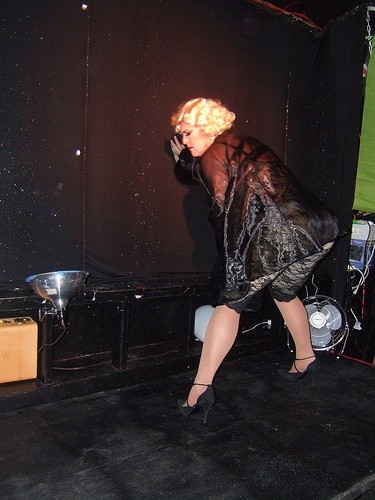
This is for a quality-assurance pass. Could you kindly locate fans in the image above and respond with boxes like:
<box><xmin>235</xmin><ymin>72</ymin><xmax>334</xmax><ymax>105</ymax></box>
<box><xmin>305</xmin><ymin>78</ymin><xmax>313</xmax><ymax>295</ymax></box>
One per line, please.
<box><xmin>286</xmin><ymin>294</ymin><xmax>351</xmax><ymax>359</ymax></box>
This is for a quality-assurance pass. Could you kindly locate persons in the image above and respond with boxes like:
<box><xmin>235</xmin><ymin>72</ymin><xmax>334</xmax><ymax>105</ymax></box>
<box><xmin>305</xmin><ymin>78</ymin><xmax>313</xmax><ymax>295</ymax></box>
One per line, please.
<box><xmin>168</xmin><ymin>96</ymin><xmax>354</xmax><ymax>425</ymax></box>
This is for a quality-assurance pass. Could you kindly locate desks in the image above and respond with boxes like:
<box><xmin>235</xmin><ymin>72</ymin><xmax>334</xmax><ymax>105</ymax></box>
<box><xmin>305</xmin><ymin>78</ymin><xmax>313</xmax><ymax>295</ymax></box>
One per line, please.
<box><xmin>0</xmin><ymin>273</ymin><xmax>281</xmax><ymax>387</ymax></box>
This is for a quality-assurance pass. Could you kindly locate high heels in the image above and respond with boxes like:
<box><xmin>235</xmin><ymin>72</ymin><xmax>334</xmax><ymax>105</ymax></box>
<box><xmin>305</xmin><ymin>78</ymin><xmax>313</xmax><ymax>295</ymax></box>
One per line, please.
<box><xmin>276</xmin><ymin>355</ymin><xmax>322</xmax><ymax>387</ymax></box>
<box><xmin>175</xmin><ymin>382</ymin><xmax>216</xmax><ymax>425</ymax></box>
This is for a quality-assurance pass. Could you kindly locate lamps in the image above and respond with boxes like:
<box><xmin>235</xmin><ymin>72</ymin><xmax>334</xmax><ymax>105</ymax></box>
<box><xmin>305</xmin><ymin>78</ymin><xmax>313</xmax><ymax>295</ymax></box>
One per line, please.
<box><xmin>25</xmin><ymin>270</ymin><xmax>90</xmax><ymax>355</ymax></box>
